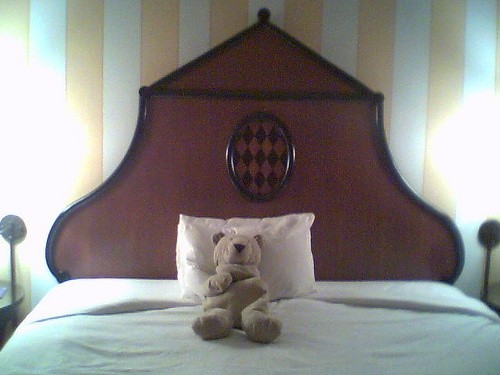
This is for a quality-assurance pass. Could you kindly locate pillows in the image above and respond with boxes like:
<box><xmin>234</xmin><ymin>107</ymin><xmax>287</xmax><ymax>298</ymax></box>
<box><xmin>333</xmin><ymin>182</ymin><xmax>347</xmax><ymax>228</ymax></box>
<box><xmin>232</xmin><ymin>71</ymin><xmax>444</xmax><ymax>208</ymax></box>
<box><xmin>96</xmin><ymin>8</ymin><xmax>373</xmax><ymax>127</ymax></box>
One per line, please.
<box><xmin>177</xmin><ymin>213</ymin><xmax>316</xmax><ymax>304</ymax></box>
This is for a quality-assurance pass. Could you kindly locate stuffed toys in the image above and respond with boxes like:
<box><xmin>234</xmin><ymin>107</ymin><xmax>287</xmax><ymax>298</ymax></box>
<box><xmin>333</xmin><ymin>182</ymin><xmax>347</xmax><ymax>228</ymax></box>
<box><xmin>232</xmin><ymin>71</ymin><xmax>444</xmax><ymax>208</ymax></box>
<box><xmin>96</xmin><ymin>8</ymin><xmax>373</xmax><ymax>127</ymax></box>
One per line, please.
<box><xmin>192</xmin><ymin>231</ymin><xmax>281</xmax><ymax>344</ymax></box>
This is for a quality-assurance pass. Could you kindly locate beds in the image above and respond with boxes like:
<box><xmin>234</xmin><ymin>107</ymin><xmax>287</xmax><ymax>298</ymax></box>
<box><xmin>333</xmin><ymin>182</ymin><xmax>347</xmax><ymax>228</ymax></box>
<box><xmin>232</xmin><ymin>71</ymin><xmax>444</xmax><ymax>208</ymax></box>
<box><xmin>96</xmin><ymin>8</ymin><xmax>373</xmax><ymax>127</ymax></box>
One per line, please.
<box><xmin>0</xmin><ymin>276</ymin><xmax>498</xmax><ymax>374</ymax></box>
